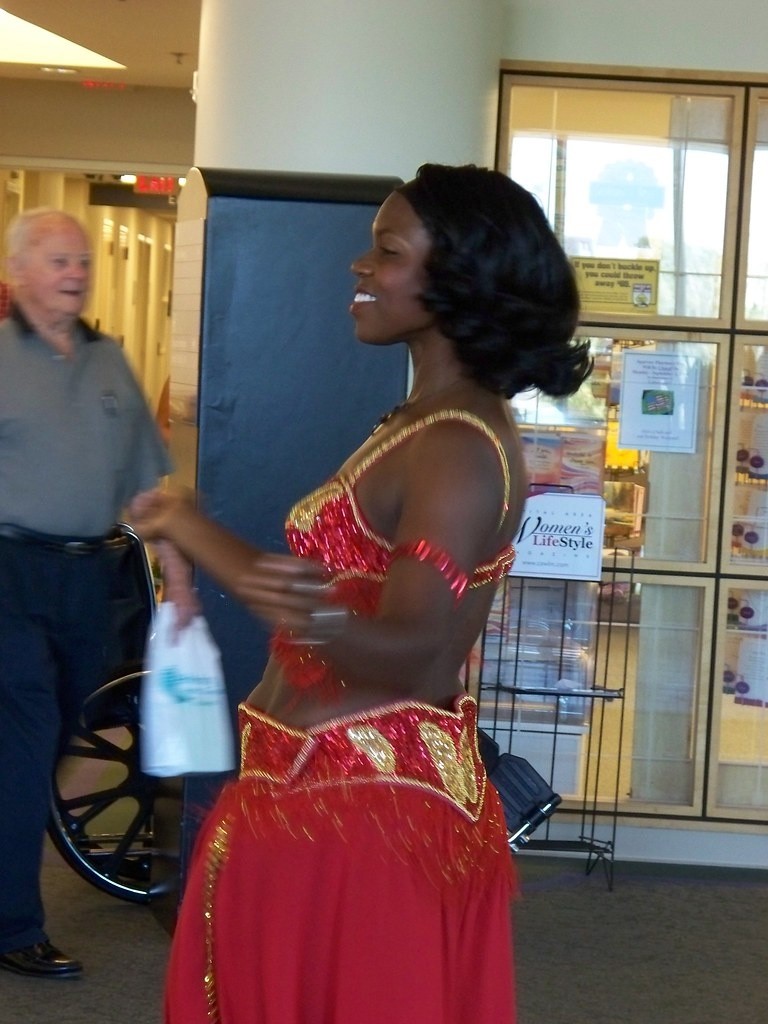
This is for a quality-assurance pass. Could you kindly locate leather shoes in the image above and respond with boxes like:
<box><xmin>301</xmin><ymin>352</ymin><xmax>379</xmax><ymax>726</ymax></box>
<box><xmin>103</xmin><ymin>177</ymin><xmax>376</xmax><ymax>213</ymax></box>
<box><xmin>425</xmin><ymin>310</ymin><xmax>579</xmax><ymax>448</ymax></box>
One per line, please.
<box><xmin>0</xmin><ymin>939</ymin><xmax>84</xmax><ymax>978</ymax></box>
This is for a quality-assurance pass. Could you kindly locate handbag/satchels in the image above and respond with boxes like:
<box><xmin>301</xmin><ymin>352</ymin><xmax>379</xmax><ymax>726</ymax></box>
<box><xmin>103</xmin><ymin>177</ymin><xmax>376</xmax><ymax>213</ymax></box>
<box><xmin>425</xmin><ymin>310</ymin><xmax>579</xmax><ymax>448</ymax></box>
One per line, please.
<box><xmin>139</xmin><ymin>602</ymin><xmax>235</xmax><ymax>777</ymax></box>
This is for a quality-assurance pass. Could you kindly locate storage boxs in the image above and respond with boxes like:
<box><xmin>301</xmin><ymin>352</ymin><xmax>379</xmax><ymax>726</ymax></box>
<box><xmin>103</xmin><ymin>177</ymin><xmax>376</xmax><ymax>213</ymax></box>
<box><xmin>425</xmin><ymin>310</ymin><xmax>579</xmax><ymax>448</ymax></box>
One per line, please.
<box><xmin>460</xmin><ymin>491</ymin><xmax>612</xmax><ymax>796</ymax></box>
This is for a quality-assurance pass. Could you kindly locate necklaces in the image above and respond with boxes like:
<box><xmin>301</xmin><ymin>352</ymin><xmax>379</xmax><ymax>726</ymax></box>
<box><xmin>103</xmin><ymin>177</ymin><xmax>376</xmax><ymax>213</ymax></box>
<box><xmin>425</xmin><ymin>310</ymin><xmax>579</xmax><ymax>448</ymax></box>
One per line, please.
<box><xmin>370</xmin><ymin>371</ymin><xmax>474</xmax><ymax>435</ymax></box>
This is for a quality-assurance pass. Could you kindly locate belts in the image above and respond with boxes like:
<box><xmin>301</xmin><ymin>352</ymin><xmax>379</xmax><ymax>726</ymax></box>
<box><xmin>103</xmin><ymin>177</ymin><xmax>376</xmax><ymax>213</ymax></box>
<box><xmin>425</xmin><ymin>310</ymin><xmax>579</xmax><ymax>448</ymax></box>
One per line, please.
<box><xmin>0</xmin><ymin>524</ymin><xmax>128</xmax><ymax>555</ymax></box>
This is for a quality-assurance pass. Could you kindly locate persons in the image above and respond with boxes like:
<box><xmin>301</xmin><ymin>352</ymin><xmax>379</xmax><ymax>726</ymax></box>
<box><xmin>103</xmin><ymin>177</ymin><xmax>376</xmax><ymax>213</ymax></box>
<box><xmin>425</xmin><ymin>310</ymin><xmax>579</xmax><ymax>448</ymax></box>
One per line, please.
<box><xmin>0</xmin><ymin>205</ymin><xmax>199</xmax><ymax>979</ymax></box>
<box><xmin>119</xmin><ymin>162</ymin><xmax>595</xmax><ymax>1024</ymax></box>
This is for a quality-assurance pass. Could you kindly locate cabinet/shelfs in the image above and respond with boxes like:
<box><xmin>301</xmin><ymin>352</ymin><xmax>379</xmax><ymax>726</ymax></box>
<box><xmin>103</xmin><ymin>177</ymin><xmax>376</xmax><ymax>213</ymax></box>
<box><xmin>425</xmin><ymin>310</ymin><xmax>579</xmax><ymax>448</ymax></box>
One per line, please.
<box><xmin>465</xmin><ymin>546</ymin><xmax>637</xmax><ymax>890</ymax></box>
<box><xmin>597</xmin><ymin>403</ymin><xmax>649</xmax><ymax>625</ymax></box>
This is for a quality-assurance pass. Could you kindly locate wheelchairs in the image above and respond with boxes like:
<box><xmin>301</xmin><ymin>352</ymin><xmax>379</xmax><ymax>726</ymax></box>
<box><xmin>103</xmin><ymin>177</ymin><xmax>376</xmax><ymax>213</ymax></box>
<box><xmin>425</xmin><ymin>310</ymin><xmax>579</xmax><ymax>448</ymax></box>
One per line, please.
<box><xmin>44</xmin><ymin>520</ymin><xmax>563</xmax><ymax>907</ymax></box>
